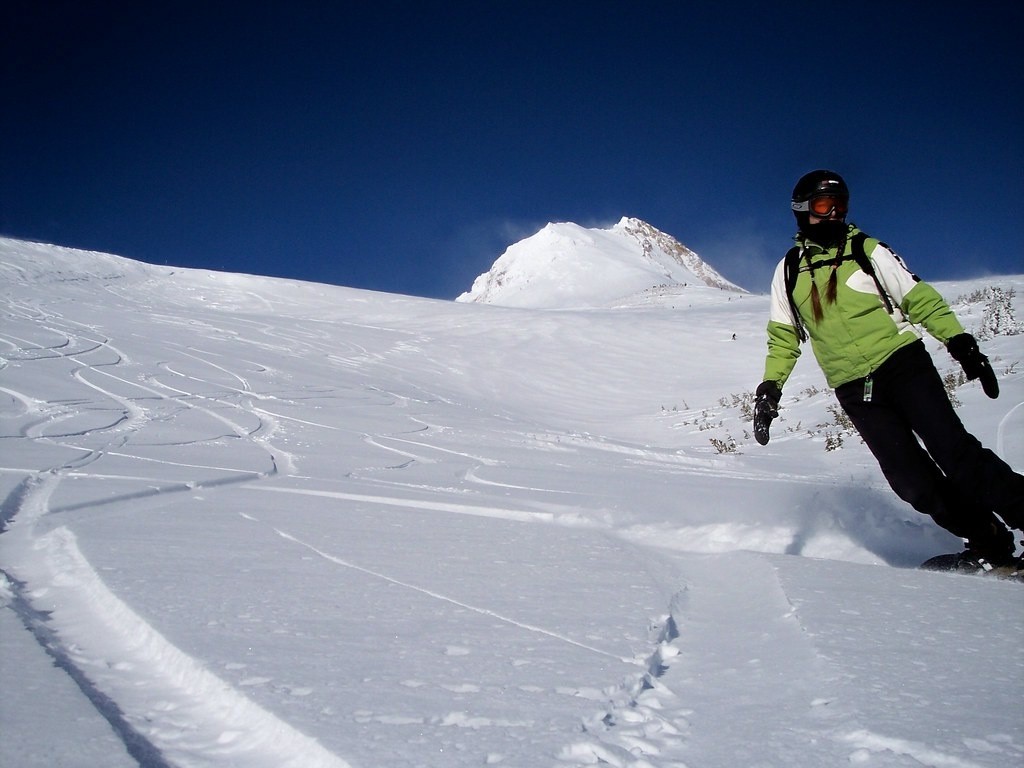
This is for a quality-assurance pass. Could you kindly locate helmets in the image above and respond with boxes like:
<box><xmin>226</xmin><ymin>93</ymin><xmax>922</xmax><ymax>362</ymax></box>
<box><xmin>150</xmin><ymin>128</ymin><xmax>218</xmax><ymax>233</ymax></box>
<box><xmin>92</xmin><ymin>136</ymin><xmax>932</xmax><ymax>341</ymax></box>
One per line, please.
<box><xmin>791</xmin><ymin>170</ymin><xmax>848</xmax><ymax>247</ymax></box>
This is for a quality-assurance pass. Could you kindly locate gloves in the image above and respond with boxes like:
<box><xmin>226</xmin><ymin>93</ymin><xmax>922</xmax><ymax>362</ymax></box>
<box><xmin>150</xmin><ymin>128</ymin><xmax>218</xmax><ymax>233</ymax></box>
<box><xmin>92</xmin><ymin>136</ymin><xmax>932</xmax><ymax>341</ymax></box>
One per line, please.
<box><xmin>753</xmin><ymin>380</ymin><xmax>782</xmax><ymax>445</ymax></box>
<box><xmin>947</xmin><ymin>333</ymin><xmax>999</xmax><ymax>399</ymax></box>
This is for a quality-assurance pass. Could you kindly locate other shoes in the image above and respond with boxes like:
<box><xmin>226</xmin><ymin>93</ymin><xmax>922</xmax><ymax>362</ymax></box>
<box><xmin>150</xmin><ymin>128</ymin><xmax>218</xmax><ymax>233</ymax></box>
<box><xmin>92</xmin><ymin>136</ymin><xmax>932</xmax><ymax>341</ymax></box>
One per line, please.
<box><xmin>952</xmin><ymin>549</ymin><xmax>1012</xmax><ymax>578</ymax></box>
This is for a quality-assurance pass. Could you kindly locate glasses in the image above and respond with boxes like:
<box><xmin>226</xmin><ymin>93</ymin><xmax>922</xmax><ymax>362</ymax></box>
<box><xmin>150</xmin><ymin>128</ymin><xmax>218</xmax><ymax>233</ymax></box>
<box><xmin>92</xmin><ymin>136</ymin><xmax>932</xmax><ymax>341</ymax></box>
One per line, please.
<box><xmin>791</xmin><ymin>193</ymin><xmax>849</xmax><ymax>218</ymax></box>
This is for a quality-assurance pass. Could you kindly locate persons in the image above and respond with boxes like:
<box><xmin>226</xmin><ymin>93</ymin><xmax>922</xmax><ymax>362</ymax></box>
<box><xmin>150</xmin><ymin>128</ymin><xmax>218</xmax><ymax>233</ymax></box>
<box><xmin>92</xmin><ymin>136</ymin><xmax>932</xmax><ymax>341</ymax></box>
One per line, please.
<box><xmin>754</xmin><ymin>169</ymin><xmax>1024</xmax><ymax>574</ymax></box>
